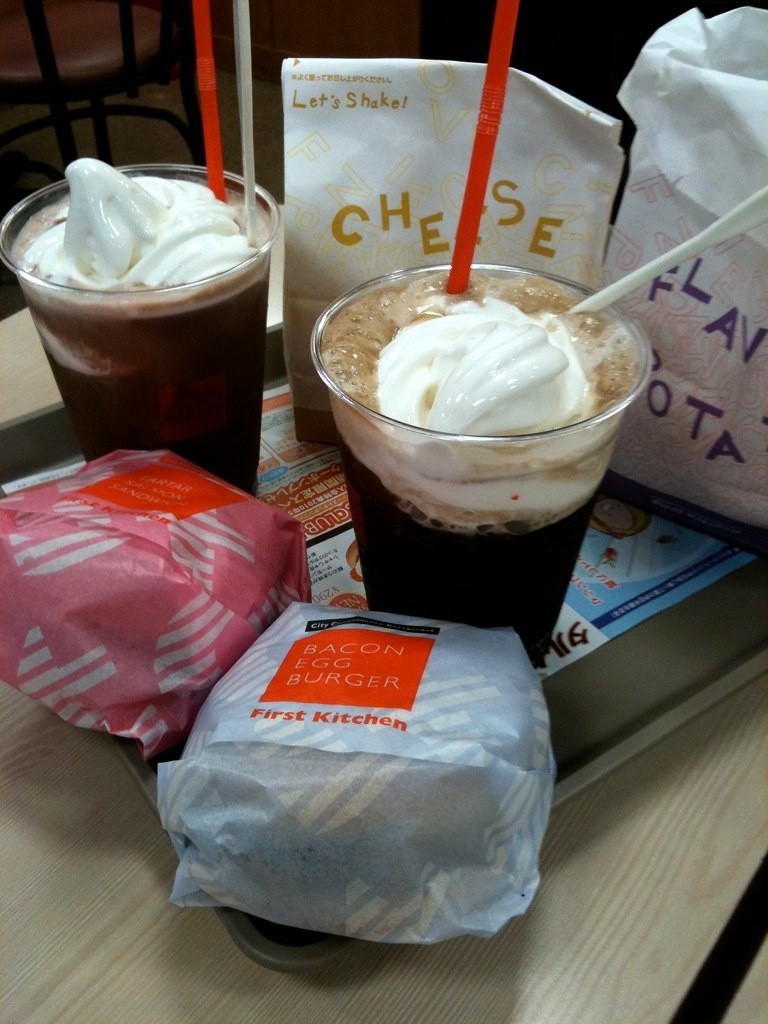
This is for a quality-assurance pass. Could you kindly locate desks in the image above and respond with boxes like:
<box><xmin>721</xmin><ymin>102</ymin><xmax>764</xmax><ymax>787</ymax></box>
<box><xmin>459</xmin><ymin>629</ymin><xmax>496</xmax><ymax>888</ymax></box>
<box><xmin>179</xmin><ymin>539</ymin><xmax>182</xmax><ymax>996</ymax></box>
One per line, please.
<box><xmin>0</xmin><ymin>202</ymin><xmax>768</xmax><ymax>1024</ymax></box>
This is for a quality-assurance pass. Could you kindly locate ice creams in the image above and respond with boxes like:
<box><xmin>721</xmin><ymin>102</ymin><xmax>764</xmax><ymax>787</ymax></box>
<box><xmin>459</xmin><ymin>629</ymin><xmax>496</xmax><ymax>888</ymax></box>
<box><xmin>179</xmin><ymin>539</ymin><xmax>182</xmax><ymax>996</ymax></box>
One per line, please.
<box><xmin>321</xmin><ymin>262</ymin><xmax>642</xmax><ymax>535</ymax></box>
<box><xmin>17</xmin><ymin>157</ymin><xmax>270</xmax><ymax>373</ymax></box>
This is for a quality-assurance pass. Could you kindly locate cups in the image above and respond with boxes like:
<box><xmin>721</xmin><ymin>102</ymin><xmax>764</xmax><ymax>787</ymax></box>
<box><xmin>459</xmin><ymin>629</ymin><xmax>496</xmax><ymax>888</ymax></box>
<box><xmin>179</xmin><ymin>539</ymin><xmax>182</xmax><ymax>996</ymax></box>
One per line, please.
<box><xmin>309</xmin><ymin>261</ymin><xmax>654</xmax><ymax>671</ymax></box>
<box><xmin>0</xmin><ymin>161</ymin><xmax>282</xmax><ymax>497</ymax></box>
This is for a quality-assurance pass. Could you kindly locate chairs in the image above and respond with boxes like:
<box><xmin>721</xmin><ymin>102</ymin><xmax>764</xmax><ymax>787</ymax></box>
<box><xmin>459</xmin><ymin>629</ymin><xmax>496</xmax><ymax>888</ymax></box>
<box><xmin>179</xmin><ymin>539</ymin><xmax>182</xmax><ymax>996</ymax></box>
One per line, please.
<box><xmin>0</xmin><ymin>0</ymin><xmax>207</xmax><ymax>188</ymax></box>
